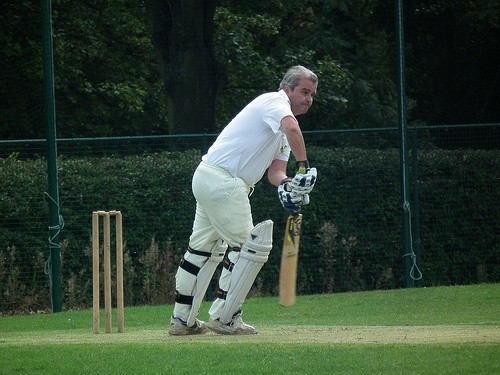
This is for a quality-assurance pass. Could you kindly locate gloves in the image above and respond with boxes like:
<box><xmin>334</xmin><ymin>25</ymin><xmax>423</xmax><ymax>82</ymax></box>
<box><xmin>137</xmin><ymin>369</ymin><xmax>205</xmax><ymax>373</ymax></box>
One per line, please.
<box><xmin>277</xmin><ymin>167</ymin><xmax>318</xmax><ymax>214</ymax></box>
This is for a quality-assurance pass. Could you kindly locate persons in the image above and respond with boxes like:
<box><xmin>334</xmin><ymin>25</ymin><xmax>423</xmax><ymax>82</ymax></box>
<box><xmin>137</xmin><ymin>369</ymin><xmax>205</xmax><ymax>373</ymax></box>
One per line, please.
<box><xmin>166</xmin><ymin>65</ymin><xmax>319</xmax><ymax>336</ymax></box>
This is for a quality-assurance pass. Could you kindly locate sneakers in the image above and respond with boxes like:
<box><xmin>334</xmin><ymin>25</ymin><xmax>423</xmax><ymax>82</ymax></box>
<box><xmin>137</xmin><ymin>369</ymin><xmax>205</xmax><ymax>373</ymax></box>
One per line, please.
<box><xmin>167</xmin><ymin>316</ymin><xmax>209</xmax><ymax>335</ymax></box>
<box><xmin>208</xmin><ymin>313</ymin><xmax>258</xmax><ymax>334</ymax></box>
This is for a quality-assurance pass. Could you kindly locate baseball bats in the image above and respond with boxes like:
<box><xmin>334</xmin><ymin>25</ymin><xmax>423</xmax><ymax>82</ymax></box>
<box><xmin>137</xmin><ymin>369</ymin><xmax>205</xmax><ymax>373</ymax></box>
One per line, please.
<box><xmin>279</xmin><ymin>166</ymin><xmax>306</xmax><ymax>308</ymax></box>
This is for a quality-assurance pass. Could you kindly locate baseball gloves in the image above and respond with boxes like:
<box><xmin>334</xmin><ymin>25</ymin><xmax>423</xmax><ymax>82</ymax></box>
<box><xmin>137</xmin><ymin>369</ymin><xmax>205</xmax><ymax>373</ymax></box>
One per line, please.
<box><xmin>278</xmin><ymin>160</ymin><xmax>317</xmax><ymax>213</ymax></box>
